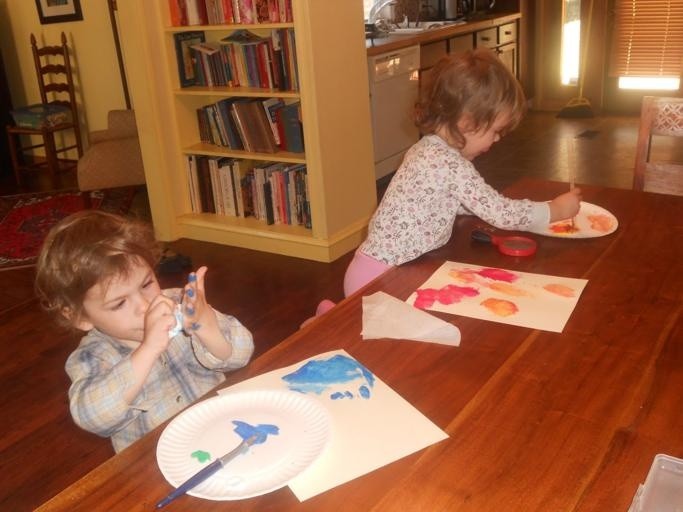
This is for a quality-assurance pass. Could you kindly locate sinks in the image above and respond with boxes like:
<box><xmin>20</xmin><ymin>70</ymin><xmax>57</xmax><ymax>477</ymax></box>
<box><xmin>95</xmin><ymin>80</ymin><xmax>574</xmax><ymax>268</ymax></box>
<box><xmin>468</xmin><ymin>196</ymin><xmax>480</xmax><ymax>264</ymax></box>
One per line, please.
<box><xmin>389</xmin><ymin>21</ymin><xmax>468</xmax><ymax>30</ymax></box>
<box><xmin>389</xmin><ymin>28</ymin><xmax>424</xmax><ymax>34</ymax></box>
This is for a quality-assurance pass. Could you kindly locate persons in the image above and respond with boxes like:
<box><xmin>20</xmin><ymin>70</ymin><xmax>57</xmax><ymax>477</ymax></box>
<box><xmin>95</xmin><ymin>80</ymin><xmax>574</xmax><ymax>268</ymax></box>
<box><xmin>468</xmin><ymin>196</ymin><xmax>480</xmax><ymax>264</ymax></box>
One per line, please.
<box><xmin>32</xmin><ymin>210</ymin><xmax>255</xmax><ymax>455</ymax></box>
<box><xmin>297</xmin><ymin>49</ymin><xmax>583</xmax><ymax>330</ymax></box>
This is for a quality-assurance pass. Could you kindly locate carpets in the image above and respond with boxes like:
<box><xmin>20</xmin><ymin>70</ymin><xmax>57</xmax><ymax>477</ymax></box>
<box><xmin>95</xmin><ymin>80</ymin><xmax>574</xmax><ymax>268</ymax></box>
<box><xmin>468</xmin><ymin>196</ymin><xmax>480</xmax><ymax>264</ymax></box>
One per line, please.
<box><xmin>0</xmin><ymin>183</ymin><xmax>136</xmax><ymax>271</ymax></box>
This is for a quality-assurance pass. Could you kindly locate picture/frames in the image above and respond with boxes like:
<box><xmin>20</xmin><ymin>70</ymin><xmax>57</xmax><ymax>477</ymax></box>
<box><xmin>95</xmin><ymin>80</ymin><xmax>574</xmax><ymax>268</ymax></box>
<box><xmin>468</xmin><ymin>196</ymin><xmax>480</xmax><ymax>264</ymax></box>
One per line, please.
<box><xmin>35</xmin><ymin>0</ymin><xmax>84</xmax><ymax>25</ymax></box>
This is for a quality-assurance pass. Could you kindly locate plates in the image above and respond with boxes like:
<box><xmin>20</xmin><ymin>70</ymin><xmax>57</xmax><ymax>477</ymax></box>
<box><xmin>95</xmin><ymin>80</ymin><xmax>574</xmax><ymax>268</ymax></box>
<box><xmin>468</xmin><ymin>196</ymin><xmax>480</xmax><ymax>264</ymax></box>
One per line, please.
<box><xmin>155</xmin><ymin>388</ymin><xmax>333</xmax><ymax>501</ymax></box>
<box><xmin>525</xmin><ymin>199</ymin><xmax>618</xmax><ymax>238</ymax></box>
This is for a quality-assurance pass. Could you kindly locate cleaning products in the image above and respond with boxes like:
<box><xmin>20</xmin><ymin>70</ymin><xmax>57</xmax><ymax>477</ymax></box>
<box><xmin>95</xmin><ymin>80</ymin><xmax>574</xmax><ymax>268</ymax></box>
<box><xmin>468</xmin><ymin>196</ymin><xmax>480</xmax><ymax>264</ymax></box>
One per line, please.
<box><xmin>444</xmin><ymin>0</ymin><xmax>457</xmax><ymax>19</ymax></box>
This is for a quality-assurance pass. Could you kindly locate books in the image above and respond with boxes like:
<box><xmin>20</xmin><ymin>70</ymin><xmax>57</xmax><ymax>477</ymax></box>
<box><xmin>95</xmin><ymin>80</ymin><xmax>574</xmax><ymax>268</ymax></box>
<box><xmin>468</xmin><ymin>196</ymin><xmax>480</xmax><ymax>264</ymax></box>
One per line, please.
<box><xmin>169</xmin><ymin>2</ymin><xmax>313</xmax><ymax>230</ymax></box>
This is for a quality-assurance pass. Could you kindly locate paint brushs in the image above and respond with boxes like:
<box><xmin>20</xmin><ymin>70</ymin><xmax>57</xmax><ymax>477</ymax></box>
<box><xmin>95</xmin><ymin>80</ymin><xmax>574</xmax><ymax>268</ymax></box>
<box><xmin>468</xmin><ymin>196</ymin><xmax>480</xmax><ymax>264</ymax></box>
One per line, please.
<box><xmin>157</xmin><ymin>434</ymin><xmax>258</xmax><ymax>508</ymax></box>
<box><xmin>567</xmin><ymin>136</ymin><xmax>575</xmax><ymax>228</ymax></box>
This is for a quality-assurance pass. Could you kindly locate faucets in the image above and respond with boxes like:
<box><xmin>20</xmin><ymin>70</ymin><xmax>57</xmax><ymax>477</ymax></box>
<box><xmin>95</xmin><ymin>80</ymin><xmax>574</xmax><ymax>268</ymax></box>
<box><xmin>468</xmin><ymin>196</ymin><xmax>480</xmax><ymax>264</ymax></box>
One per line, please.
<box><xmin>369</xmin><ymin>0</ymin><xmax>398</xmax><ymax>23</ymax></box>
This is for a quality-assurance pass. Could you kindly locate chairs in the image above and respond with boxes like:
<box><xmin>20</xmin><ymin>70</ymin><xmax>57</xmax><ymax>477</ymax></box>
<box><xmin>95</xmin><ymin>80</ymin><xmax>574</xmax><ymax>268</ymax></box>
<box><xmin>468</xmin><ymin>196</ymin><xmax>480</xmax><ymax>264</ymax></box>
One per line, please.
<box><xmin>632</xmin><ymin>95</ymin><xmax>683</xmax><ymax>197</ymax></box>
<box><xmin>8</xmin><ymin>32</ymin><xmax>84</xmax><ymax>189</ymax></box>
<box><xmin>77</xmin><ymin>109</ymin><xmax>145</xmax><ymax>209</ymax></box>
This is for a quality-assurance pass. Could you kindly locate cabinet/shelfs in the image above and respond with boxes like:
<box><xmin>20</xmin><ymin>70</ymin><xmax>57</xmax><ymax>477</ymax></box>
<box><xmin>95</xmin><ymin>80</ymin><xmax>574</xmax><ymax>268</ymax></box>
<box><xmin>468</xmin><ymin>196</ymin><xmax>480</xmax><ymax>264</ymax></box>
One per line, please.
<box><xmin>420</xmin><ymin>22</ymin><xmax>517</xmax><ymax>105</ymax></box>
<box><xmin>114</xmin><ymin>0</ymin><xmax>376</xmax><ymax>262</ymax></box>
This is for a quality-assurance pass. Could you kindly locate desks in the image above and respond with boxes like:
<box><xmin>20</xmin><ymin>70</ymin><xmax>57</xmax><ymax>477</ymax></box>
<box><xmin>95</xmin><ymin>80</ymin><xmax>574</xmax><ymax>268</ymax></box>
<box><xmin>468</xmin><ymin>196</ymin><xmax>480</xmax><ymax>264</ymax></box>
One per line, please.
<box><xmin>30</xmin><ymin>175</ymin><xmax>683</xmax><ymax>512</ymax></box>
